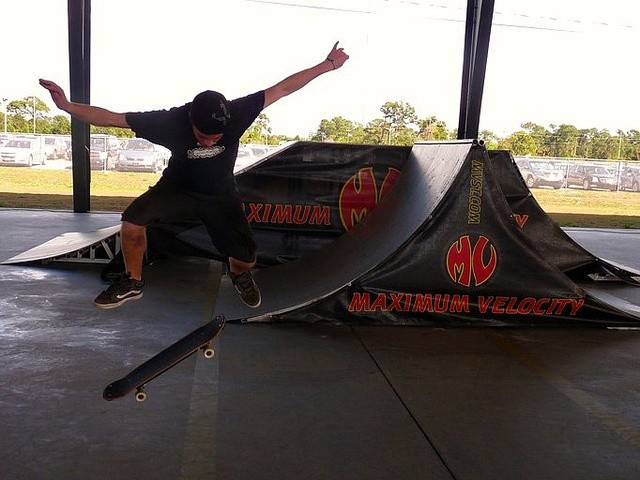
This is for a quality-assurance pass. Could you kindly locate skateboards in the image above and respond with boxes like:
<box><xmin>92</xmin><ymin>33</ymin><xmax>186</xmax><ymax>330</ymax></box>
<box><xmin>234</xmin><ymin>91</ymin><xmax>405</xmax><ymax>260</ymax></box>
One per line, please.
<box><xmin>103</xmin><ymin>314</ymin><xmax>226</xmax><ymax>402</ymax></box>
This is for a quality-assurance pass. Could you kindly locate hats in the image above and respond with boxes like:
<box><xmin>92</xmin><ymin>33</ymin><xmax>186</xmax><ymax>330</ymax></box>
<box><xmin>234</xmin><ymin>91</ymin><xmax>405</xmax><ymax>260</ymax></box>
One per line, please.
<box><xmin>191</xmin><ymin>90</ymin><xmax>232</xmax><ymax>136</ymax></box>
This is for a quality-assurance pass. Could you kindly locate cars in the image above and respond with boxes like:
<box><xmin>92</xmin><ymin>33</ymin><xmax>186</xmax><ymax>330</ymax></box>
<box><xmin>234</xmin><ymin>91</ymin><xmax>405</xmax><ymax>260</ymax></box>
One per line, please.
<box><xmin>235</xmin><ymin>147</ymin><xmax>266</xmax><ymax>166</ymax></box>
<box><xmin>619</xmin><ymin>165</ymin><xmax>640</xmax><ymax>192</ymax></box>
<box><xmin>566</xmin><ymin>164</ymin><xmax>618</xmax><ymax>191</ymax></box>
<box><xmin>554</xmin><ymin>162</ymin><xmax>567</xmax><ymax>178</ymax></box>
<box><xmin>114</xmin><ymin>138</ymin><xmax>163</xmax><ymax>173</ymax></box>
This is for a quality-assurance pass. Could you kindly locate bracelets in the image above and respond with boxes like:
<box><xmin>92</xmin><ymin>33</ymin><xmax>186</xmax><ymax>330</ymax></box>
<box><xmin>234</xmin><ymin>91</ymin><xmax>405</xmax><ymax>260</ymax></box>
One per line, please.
<box><xmin>326</xmin><ymin>57</ymin><xmax>335</xmax><ymax>70</ymax></box>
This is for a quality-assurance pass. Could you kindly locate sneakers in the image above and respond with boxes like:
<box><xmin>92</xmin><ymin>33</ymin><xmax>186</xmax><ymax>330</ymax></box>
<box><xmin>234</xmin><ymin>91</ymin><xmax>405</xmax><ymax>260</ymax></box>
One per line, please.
<box><xmin>93</xmin><ymin>271</ymin><xmax>145</xmax><ymax>310</ymax></box>
<box><xmin>226</xmin><ymin>265</ymin><xmax>261</xmax><ymax>308</ymax></box>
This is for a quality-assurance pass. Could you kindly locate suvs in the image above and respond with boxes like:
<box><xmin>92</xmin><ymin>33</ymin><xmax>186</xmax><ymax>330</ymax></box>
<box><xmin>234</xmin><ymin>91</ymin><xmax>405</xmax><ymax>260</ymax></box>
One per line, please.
<box><xmin>0</xmin><ymin>134</ymin><xmax>47</xmax><ymax>167</ymax></box>
<box><xmin>516</xmin><ymin>158</ymin><xmax>564</xmax><ymax>189</ymax></box>
<box><xmin>87</xmin><ymin>133</ymin><xmax>120</xmax><ymax>171</ymax></box>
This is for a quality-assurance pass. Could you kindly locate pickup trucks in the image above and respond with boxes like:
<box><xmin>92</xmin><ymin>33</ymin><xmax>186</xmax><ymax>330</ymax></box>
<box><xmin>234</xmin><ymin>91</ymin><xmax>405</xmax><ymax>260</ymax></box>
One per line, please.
<box><xmin>44</xmin><ymin>136</ymin><xmax>68</xmax><ymax>160</ymax></box>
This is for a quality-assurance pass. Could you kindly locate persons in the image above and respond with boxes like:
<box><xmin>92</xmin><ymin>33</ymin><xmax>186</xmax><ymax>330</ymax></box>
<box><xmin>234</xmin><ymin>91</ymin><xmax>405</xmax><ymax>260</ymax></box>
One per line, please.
<box><xmin>37</xmin><ymin>39</ymin><xmax>350</xmax><ymax>309</ymax></box>
